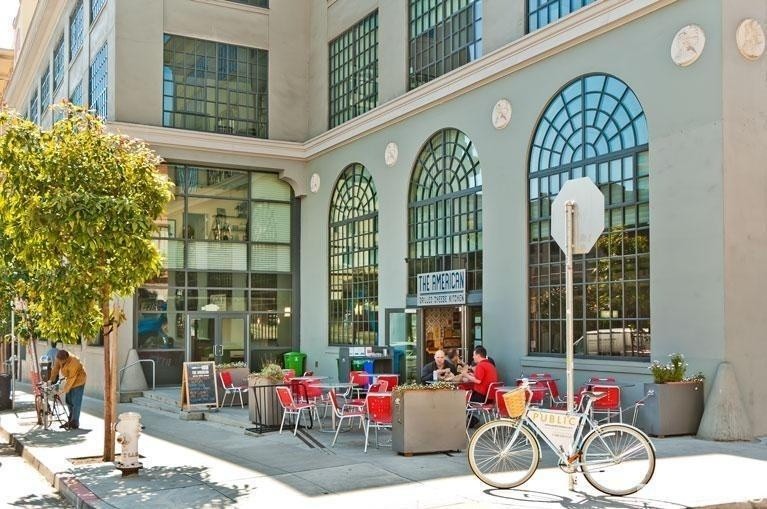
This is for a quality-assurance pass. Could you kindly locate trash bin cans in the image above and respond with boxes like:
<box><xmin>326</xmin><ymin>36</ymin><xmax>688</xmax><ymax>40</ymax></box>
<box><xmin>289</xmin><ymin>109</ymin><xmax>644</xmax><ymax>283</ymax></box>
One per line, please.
<box><xmin>282</xmin><ymin>352</ymin><xmax>307</xmax><ymax>376</ymax></box>
<box><xmin>0</xmin><ymin>372</ymin><xmax>12</xmax><ymax>409</ymax></box>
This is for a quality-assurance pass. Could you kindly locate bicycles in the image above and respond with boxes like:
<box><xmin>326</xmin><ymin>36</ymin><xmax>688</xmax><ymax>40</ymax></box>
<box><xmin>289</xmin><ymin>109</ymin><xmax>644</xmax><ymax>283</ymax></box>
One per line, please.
<box><xmin>464</xmin><ymin>377</ymin><xmax>656</xmax><ymax>498</ymax></box>
<box><xmin>34</xmin><ymin>375</ymin><xmax>71</xmax><ymax>432</ymax></box>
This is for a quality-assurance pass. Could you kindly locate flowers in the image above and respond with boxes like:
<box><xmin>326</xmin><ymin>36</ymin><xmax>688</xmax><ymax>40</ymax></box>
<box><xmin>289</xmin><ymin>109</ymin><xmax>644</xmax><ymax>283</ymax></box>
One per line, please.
<box><xmin>647</xmin><ymin>350</ymin><xmax>705</xmax><ymax>385</ymax></box>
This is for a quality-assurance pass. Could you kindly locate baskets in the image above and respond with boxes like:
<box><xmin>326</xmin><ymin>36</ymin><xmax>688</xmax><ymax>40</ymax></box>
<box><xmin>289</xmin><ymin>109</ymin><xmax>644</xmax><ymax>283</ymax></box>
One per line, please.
<box><xmin>501</xmin><ymin>386</ymin><xmax>527</xmax><ymax>418</ymax></box>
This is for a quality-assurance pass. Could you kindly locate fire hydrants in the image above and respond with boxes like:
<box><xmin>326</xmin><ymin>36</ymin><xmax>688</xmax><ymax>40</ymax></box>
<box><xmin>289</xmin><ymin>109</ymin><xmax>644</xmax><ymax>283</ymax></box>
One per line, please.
<box><xmin>110</xmin><ymin>411</ymin><xmax>145</xmax><ymax>478</ymax></box>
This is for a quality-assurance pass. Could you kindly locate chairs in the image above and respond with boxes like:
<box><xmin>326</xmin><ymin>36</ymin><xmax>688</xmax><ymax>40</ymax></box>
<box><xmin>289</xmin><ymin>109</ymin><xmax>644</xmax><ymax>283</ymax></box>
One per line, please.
<box><xmin>276</xmin><ymin>369</ymin><xmax>399</xmax><ymax>452</ymax></box>
<box><xmin>574</xmin><ymin>376</ymin><xmax>636</xmax><ymax>436</ymax></box>
<box><xmin>465</xmin><ymin>373</ymin><xmax>568</xmax><ymax>444</ymax></box>
<box><xmin>218</xmin><ymin>371</ymin><xmax>247</xmax><ymax>408</ymax></box>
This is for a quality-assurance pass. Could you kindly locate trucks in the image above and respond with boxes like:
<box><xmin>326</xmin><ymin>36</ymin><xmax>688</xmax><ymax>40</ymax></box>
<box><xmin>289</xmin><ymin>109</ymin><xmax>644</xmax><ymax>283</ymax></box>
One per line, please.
<box><xmin>573</xmin><ymin>328</ymin><xmax>632</xmax><ymax>356</ymax></box>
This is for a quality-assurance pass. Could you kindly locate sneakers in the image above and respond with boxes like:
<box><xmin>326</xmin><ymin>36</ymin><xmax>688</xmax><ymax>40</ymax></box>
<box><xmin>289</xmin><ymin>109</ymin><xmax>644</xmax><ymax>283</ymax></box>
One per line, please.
<box><xmin>59</xmin><ymin>420</ymin><xmax>80</xmax><ymax>432</ymax></box>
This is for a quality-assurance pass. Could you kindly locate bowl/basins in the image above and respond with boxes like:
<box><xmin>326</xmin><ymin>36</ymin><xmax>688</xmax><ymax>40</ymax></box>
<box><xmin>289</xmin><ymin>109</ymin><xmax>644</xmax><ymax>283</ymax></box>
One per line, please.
<box><xmin>463</xmin><ymin>378</ymin><xmax>470</xmax><ymax>382</ymax></box>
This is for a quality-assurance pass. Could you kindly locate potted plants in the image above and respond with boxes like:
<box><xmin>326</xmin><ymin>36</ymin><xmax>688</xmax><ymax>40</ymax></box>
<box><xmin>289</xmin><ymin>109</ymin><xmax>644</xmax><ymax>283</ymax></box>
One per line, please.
<box><xmin>215</xmin><ymin>360</ymin><xmax>249</xmax><ymax>405</ymax></box>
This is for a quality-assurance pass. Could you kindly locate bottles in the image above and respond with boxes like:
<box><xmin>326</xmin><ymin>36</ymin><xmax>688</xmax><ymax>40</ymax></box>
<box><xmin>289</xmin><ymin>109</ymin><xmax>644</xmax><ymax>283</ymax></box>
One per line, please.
<box><xmin>520</xmin><ymin>370</ymin><xmax>524</xmax><ymax>378</ymax></box>
<box><xmin>371</xmin><ymin>349</ymin><xmax>387</xmax><ymax>359</ymax></box>
<box><xmin>433</xmin><ymin>371</ymin><xmax>438</xmax><ymax>381</ymax></box>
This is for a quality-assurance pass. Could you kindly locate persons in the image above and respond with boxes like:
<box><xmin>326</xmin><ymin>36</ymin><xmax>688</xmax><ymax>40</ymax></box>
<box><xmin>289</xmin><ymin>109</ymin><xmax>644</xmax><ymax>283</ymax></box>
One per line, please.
<box><xmin>461</xmin><ymin>348</ymin><xmax>499</xmax><ymax>427</ymax></box>
<box><xmin>446</xmin><ymin>348</ymin><xmax>465</xmax><ymax>375</ymax></box>
<box><xmin>43</xmin><ymin>341</ymin><xmax>60</xmax><ymax>384</ymax></box>
<box><xmin>47</xmin><ymin>350</ymin><xmax>87</xmax><ymax>430</ymax></box>
<box><xmin>468</xmin><ymin>345</ymin><xmax>496</xmax><ymax>373</ymax></box>
<box><xmin>421</xmin><ymin>350</ymin><xmax>458</xmax><ymax>385</ymax></box>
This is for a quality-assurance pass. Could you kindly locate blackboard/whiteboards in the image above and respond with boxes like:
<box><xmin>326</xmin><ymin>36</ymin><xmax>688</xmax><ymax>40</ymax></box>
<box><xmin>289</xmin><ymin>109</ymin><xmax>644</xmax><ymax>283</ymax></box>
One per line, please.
<box><xmin>184</xmin><ymin>361</ymin><xmax>217</xmax><ymax>406</ymax></box>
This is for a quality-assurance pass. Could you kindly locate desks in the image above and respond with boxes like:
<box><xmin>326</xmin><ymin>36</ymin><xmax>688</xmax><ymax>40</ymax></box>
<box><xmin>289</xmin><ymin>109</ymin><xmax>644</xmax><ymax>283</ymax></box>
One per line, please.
<box><xmin>427</xmin><ymin>380</ymin><xmax>464</xmax><ymax>384</ymax></box>
